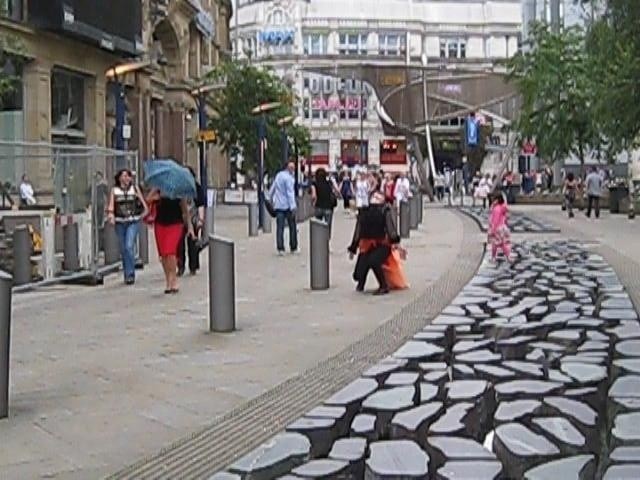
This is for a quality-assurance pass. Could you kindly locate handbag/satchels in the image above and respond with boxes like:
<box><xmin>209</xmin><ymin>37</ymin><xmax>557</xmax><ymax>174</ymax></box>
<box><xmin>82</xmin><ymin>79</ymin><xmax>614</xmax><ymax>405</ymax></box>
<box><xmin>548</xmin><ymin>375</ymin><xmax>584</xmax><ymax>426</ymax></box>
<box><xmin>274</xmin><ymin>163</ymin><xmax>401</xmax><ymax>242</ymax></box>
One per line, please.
<box><xmin>133</xmin><ymin>198</ymin><xmax>143</xmax><ymax>214</ymax></box>
<box><xmin>329</xmin><ymin>192</ymin><xmax>337</xmax><ymax>207</ymax></box>
<box><xmin>193</xmin><ymin>218</ymin><xmax>209</xmax><ymax>253</ymax></box>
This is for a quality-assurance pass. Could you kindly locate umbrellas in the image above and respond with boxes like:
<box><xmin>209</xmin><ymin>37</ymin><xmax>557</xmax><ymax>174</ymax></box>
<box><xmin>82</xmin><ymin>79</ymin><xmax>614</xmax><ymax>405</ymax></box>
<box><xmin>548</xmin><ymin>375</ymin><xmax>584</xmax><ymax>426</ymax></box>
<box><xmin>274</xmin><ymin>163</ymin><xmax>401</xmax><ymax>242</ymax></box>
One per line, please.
<box><xmin>143</xmin><ymin>158</ymin><xmax>197</xmax><ymax>199</ymax></box>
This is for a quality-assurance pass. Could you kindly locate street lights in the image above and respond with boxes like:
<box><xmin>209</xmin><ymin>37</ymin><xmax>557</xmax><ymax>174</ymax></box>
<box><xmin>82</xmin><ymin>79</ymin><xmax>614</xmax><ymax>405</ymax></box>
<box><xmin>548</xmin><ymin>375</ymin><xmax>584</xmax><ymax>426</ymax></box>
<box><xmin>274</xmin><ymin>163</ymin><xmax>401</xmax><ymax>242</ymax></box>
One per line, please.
<box><xmin>276</xmin><ymin>116</ymin><xmax>294</xmax><ymax>165</ymax></box>
<box><xmin>106</xmin><ymin>59</ymin><xmax>168</xmax><ymax>173</ymax></box>
<box><xmin>192</xmin><ymin>83</ymin><xmax>228</xmax><ymax>206</ymax></box>
<box><xmin>249</xmin><ymin>100</ymin><xmax>283</xmax><ymax>228</ymax></box>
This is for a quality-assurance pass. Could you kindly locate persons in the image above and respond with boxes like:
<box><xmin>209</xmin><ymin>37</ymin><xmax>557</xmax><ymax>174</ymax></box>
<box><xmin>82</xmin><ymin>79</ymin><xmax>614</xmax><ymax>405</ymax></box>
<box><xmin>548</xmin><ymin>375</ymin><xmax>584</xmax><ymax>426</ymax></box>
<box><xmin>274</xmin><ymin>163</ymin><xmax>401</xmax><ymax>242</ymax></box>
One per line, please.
<box><xmin>585</xmin><ymin>166</ymin><xmax>603</xmax><ymax>217</ymax></box>
<box><xmin>563</xmin><ymin>172</ymin><xmax>580</xmax><ymax>218</ymax></box>
<box><xmin>176</xmin><ymin>165</ymin><xmax>204</xmax><ymax>277</ymax></box>
<box><xmin>268</xmin><ymin>161</ymin><xmax>413</xmax><ymax>294</ymax></box>
<box><xmin>432</xmin><ymin>162</ymin><xmax>554</xmax><ymax>210</ymax></box>
<box><xmin>146</xmin><ymin>188</ymin><xmax>194</xmax><ymax>294</ymax></box>
<box><xmin>487</xmin><ymin>193</ymin><xmax>514</xmax><ymax>263</ymax></box>
<box><xmin>87</xmin><ymin>172</ymin><xmax>108</xmax><ymax>227</ymax></box>
<box><xmin>19</xmin><ymin>175</ymin><xmax>37</xmax><ymax>207</ymax></box>
<box><xmin>107</xmin><ymin>168</ymin><xmax>148</xmax><ymax>284</ymax></box>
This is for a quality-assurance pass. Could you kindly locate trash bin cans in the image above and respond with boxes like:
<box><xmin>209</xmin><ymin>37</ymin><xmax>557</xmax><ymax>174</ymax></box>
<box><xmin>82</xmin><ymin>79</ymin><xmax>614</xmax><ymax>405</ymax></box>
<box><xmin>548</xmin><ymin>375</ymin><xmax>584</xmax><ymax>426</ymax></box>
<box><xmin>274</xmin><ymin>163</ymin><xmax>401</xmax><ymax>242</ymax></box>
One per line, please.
<box><xmin>609</xmin><ymin>187</ymin><xmax>631</xmax><ymax>214</ymax></box>
